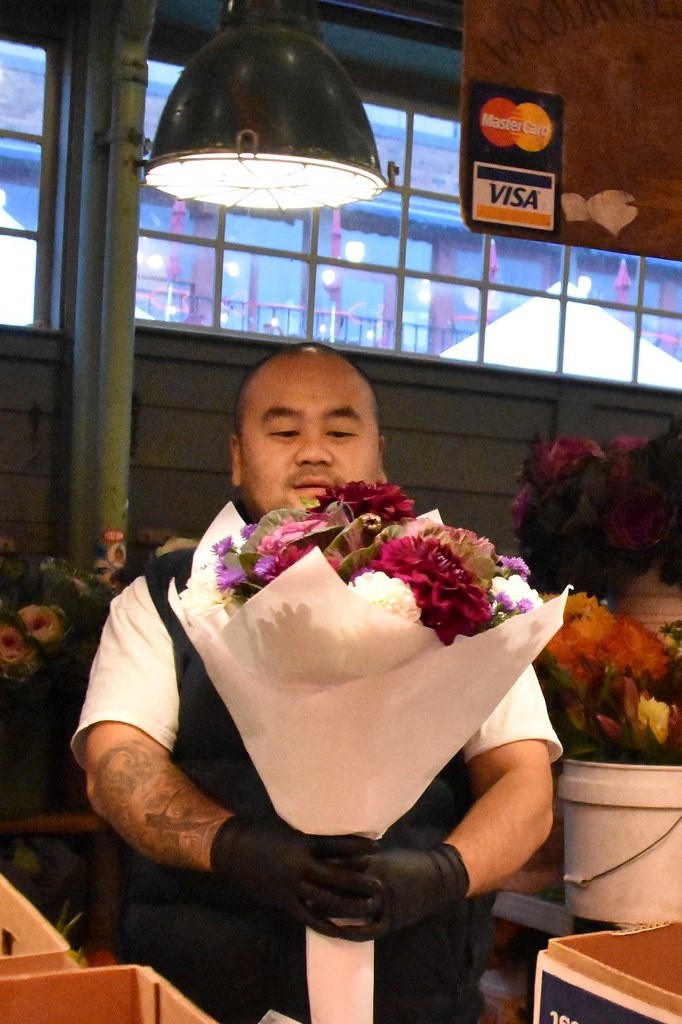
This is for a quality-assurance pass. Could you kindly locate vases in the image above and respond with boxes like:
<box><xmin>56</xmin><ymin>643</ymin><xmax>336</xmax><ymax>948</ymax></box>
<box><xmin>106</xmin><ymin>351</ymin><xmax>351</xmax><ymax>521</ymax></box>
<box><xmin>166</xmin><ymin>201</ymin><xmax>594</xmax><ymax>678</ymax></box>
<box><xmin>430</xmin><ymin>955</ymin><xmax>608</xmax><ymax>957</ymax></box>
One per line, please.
<box><xmin>558</xmin><ymin>757</ymin><xmax>681</xmax><ymax>922</ymax></box>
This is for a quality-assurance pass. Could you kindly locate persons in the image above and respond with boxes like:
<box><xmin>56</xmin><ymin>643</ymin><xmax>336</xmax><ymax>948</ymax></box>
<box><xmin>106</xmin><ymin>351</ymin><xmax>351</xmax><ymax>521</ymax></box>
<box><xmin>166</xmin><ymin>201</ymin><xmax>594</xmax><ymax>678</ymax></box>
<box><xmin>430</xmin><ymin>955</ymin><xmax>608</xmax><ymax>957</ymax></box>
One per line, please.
<box><xmin>69</xmin><ymin>340</ymin><xmax>566</xmax><ymax>1024</ymax></box>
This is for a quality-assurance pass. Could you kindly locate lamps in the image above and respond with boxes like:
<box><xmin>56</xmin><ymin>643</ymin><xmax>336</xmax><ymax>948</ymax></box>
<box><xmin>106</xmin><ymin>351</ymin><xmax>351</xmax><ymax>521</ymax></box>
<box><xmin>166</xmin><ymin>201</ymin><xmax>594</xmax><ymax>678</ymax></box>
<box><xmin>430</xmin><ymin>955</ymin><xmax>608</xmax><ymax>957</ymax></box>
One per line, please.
<box><xmin>147</xmin><ymin>1</ymin><xmax>385</xmax><ymax>207</ymax></box>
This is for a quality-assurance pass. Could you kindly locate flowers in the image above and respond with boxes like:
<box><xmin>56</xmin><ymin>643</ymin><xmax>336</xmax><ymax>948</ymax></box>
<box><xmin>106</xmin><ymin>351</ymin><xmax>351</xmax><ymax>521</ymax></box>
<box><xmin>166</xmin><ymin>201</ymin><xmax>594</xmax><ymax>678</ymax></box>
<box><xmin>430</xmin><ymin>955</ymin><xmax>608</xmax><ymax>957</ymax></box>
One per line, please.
<box><xmin>0</xmin><ymin>602</ymin><xmax>70</xmax><ymax>684</ymax></box>
<box><xmin>539</xmin><ymin>586</ymin><xmax>680</xmax><ymax>770</ymax></box>
<box><xmin>217</xmin><ymin>480</ymin><xmax>540</xmax><ymax>648</ymax></box>
<box><xmin>515</xmin><ymin>427</ymin><xmax>680</xmax><ymax>589</ymax></box>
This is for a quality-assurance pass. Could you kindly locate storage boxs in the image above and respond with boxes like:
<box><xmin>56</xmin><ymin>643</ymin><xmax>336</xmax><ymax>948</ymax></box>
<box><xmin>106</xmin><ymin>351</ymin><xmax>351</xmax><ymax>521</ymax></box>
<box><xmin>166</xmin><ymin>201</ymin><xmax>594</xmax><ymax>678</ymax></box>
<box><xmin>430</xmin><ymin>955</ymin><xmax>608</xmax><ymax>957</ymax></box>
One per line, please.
<box><xmin>1</xmin><ymin>965</ymin><xmax>220</xmax><ymax>1024</ymax></box>
<box><xmin>532</xmin><ymin>925</ymin><xmax>680</xmax><ymax>1024</ymax></box>
<box><xmin>1</xmin><ymin>874</ymin><xmax>68</xmax><ymax>974</ymax></box>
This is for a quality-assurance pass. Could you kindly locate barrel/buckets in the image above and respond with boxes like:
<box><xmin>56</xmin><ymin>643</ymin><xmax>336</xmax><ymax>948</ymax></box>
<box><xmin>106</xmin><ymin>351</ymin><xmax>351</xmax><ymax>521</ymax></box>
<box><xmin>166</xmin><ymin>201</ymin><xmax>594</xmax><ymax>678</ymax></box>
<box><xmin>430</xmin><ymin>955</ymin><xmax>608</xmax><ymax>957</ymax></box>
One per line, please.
<box><xmin>608</xmin><ymin>552</ymin><xmax>682</xmax><ymax>663</ymax></box>
<box><xmin>552</xmin><ymin>754</ymin><xmax>682</xmax><ymax>931</ymax></box>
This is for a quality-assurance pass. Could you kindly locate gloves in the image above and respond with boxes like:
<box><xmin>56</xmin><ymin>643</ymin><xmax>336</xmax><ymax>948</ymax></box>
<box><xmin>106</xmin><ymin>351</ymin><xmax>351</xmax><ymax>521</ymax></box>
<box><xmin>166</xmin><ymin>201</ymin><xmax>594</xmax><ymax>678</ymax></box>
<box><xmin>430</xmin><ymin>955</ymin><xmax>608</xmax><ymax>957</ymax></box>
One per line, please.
<box><xmin>311</xmin><ymin>842</ymin><xmax>470</xmax><ymax>941</ymax></box>
<box><xmin>211</xmin><ymin>814</ymin><xmax>385</xmax><ymax>938</ymax></box>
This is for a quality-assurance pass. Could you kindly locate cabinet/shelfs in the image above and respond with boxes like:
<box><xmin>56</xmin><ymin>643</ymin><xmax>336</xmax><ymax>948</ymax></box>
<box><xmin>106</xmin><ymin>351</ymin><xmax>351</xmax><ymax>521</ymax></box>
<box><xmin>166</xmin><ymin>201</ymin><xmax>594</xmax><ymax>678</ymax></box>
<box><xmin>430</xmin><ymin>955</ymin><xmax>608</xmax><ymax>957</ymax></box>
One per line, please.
<box><xmin>1</xmin><ymin>677</ymin><xmax>115</xmax><ymax>966</ymax></box>
